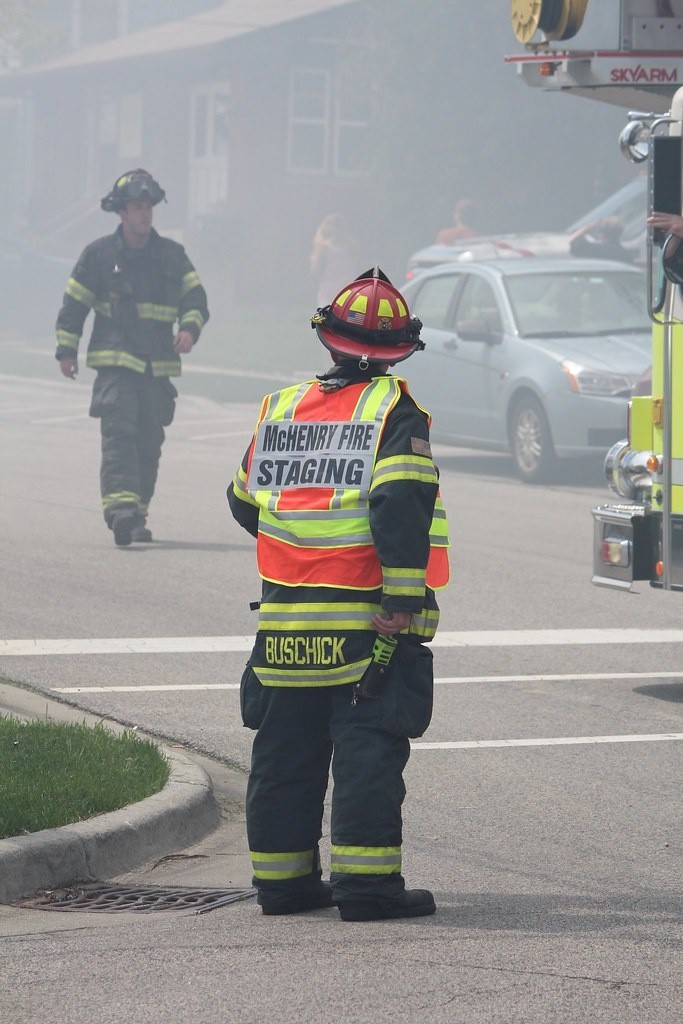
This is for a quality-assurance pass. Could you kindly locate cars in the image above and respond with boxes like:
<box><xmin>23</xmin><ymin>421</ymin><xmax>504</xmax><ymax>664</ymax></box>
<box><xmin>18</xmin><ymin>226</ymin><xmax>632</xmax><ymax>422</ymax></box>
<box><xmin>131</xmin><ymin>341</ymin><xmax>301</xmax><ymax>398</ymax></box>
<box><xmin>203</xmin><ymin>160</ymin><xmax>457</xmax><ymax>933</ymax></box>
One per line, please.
<box><xmin>385</xmin><ymin>258</ymin><xmax>652</xmax><ymax>484</ymax></box>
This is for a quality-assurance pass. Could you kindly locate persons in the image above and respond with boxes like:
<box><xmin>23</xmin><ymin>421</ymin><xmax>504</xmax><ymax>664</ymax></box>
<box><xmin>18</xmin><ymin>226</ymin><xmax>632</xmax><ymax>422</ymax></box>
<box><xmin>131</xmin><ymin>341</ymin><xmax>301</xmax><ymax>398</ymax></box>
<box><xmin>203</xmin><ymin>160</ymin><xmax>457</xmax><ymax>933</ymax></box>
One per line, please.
<box><xmin>54</xmin><ymin>169</ymin><xmax>210</xmax><ymax>547</ymax></box>
<box><xmin>435</xmin><ymin>200</ymin><xmax>485</xmax><ymax>246</ymax></box>
<box><xmin>312</xmin><ymin>213</ymin><xmax>355</xmax><ymax>308</ymax></box>
<box><xmin>226</xmin><ymin>265</ymin><xmax>451</xmax><ymax>921</ymax></box>
<box><xmin>645</xmin><ymin>211</ymin><xmax>683</xmax><ymax>237</ymax></box>
<box><xmin>568</xmin><ymin>215</ymin><xmax>639</xmax><ymax>263</ymax></box>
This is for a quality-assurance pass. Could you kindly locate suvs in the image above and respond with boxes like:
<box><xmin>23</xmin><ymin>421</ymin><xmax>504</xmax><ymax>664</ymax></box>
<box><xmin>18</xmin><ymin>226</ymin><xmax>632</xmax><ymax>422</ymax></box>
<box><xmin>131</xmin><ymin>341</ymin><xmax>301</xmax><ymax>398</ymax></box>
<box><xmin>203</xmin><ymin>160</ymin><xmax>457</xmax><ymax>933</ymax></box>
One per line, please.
<box><xmin>408</xmin><ymin>174</ymin><xmax>649</xmax><ymax>286</ymax></box>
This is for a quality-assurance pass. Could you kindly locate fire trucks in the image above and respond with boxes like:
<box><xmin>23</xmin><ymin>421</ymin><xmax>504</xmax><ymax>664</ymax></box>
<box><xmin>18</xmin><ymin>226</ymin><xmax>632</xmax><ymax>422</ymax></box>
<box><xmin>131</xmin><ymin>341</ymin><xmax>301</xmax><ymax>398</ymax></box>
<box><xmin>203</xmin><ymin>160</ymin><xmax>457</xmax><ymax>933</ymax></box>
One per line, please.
<box><xmin>503</xmin><ymin>1</ymin><xmax>683</xmax><ymax>595</ymax></box>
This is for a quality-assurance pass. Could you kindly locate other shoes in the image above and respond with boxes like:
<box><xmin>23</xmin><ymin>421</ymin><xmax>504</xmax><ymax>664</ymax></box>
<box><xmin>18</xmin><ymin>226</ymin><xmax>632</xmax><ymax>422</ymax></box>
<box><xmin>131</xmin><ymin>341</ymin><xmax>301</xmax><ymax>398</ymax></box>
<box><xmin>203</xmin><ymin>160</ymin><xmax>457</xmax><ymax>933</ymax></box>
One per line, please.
<box><xmin>261</xmin><ymin>879</ymin><xmax>337</xmax><ymax>914</ymax></box>
<box><xmin>337</xmin><ymin>889</ymin><xmax>436</xmax><ymax>921</ymax></box>
<box><xmin>112</xmin><ymin>512</ymin><xmax>132</xmax><ymax>546</ymax></box>
<box><xmin>127</xmin><ymin>517</ymin><xmax>152</xmax><ymax>542</ymax></box>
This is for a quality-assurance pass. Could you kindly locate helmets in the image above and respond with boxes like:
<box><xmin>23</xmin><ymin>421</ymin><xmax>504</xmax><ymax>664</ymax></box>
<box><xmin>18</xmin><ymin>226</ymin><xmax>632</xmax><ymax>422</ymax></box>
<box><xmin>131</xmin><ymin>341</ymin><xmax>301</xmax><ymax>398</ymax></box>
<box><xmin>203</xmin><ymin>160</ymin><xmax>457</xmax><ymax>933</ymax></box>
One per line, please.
<box><xmin>100</xmin><ymin>169</ymin><xmax>168</xmax><ymax>210</ymax></box>
<box><xmin>309</xmin><ymin>264</ymin><xmax>425</xmax><ymax>393</ymax></box>
